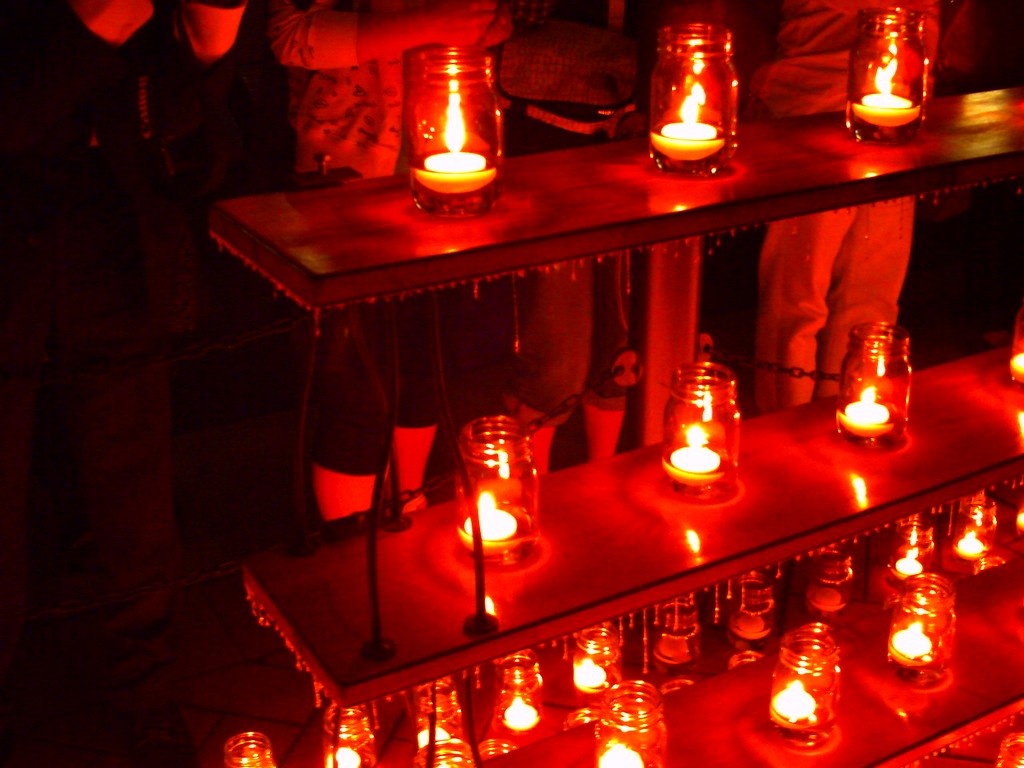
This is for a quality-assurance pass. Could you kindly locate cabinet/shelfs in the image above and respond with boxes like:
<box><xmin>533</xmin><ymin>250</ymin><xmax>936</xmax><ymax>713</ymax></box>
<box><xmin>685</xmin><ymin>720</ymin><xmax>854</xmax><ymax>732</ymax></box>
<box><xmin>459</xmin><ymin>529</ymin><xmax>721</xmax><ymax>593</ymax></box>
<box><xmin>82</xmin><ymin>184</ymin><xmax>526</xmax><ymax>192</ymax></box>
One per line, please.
<box><xmin>210</xmin><ymin>88</ymin><xmax>1024</xmax><ymax>768</ymax></box>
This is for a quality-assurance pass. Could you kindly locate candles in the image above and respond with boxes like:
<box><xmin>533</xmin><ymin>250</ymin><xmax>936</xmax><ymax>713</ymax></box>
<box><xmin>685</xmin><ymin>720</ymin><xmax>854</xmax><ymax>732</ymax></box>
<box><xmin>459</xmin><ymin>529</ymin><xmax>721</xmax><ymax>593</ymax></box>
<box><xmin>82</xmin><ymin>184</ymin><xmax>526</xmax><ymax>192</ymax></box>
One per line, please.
<box><xmin>324</xmin><ymin>60</ymin><xmax>996</xmax><ymax>768</ymax></box>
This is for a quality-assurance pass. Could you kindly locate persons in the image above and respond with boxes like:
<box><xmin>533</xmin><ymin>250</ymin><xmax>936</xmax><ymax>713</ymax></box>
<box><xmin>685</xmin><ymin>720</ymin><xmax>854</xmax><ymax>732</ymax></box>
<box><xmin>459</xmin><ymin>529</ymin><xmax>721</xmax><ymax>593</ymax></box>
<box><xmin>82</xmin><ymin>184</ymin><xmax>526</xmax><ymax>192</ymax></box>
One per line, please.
<box><xmin>265</xmin><ymin>0</ymin><xmax>1024</xmax><ymax>557</ymax></box>
<box><xmin>0</xmin><ymin>1</ymin><xmax>247</xmax><ymax>768</ymax></box>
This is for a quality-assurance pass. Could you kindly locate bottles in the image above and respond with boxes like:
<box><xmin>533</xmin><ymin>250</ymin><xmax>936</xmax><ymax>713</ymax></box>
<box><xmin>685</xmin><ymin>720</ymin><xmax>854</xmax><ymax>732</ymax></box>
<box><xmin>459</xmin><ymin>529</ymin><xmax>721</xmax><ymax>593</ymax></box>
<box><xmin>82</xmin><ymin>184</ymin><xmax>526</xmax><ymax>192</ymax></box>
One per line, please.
<box><xmin>454</xmin><ymin>415</ymin><xmax>538</xmax><ymax>561</ymax></box>
<box><xmin>407</xmin><ymin>47</ymin><xmax>504</xmax><ymax>218</ymax></box>
<box><xmin>650</xmin><ymin>24</ymin><xmax>739</xmax><ymax>176</ymax></box>
<box><xmin>663</xmin><ymin>363</ymin><xmax>743</xmax><ymax>486</ymax></box>
<box><xmin>726</xmin><ymin>548</ymin><xmax>856</xmax><ymax>746</ymax></box>
<box><xmin>847</xmin><ymin>9</ymin><xmax>930</xmax><ymax>144</ymax></box>
<box><xmin>886</xmin><ymin>496</ymin><xmax>998</xmax><ymax>670</ymax></box>
<box><xmin>837</xmin><ymin>321</ymin><xmax>911</xmax><ymax>447</ymax></box>
<box><xmin>572</xmin><ymin>599</ymin><xmax>702</xmax><ymax>768</ymax></box>
<box><xmin>223</xmin><ymin>732</ymin><xmax>278</xmax><ymax>768</ymax></box>
<box><xmin>415</xmin><ymin>655</ymin><xmax>542</xmax><ymax>768</ymax></box>
<box><xmin>321</xmin><ymin>708</ymin><xmax>379</xmax><ymax>768</ymax></box>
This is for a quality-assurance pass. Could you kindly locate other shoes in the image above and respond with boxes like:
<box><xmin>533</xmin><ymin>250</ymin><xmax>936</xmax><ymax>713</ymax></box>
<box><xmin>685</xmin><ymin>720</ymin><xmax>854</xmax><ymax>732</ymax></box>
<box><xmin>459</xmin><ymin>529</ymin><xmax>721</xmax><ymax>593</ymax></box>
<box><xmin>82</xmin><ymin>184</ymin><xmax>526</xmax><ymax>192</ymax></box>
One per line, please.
<box><xmin>125</xmin><ymin>705</ymin><xmax>196</xmax><ymax>768</ymax></box>
